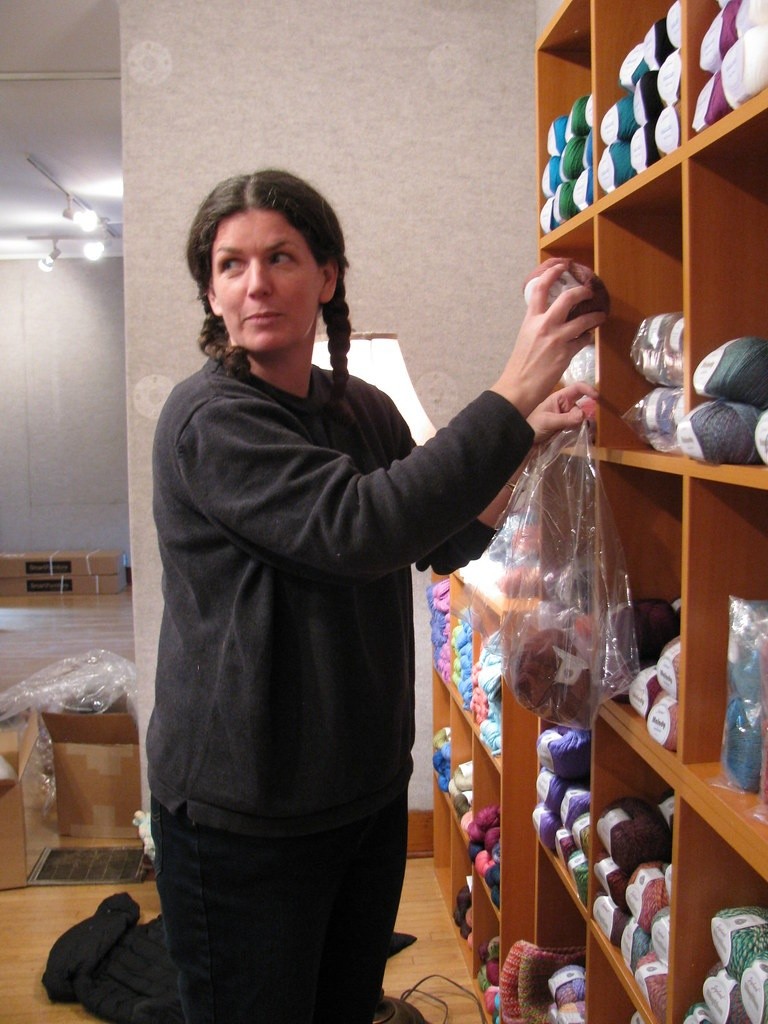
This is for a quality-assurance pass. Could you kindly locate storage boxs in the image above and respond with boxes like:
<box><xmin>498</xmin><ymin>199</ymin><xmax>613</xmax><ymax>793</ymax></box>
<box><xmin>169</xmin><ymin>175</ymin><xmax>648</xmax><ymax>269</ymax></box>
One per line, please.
<box><xmin>0</xmin><ymin>550</ymin><xmax>128</xmax><ymax>597</ymax></box>
<box><xmin>0</xmin><ymin>692</ymin><xmax>142</xmax><ymax>891</ymax></box>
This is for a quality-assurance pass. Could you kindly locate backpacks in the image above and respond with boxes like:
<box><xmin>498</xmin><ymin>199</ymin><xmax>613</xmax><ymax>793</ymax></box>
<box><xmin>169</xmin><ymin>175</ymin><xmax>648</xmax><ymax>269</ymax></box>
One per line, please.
<box><xmin>43</xmin><ymin>892</ymin><xmax>188</xmax><ymax>1024</ymax></box>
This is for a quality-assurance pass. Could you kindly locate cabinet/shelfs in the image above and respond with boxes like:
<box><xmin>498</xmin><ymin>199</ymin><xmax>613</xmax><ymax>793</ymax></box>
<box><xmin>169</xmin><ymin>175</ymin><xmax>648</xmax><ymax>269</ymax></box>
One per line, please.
<box><xmin>533</xmin><ymin>0</ymin><xmax>768</xmax><ymax>1024</ymax></box>
<box><xmin>430</xmin><ymin>557</ymin><xmax>538</xmax><ymax>1024</ymax></box>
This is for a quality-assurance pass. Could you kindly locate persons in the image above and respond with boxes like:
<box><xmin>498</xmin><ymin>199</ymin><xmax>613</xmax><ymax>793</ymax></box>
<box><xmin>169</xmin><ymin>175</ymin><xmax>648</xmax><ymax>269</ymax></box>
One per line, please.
<box><xmin>145</xmin><ymin>170</ymin><xmax>608</xmax><ymax>1024</ymax></box>
